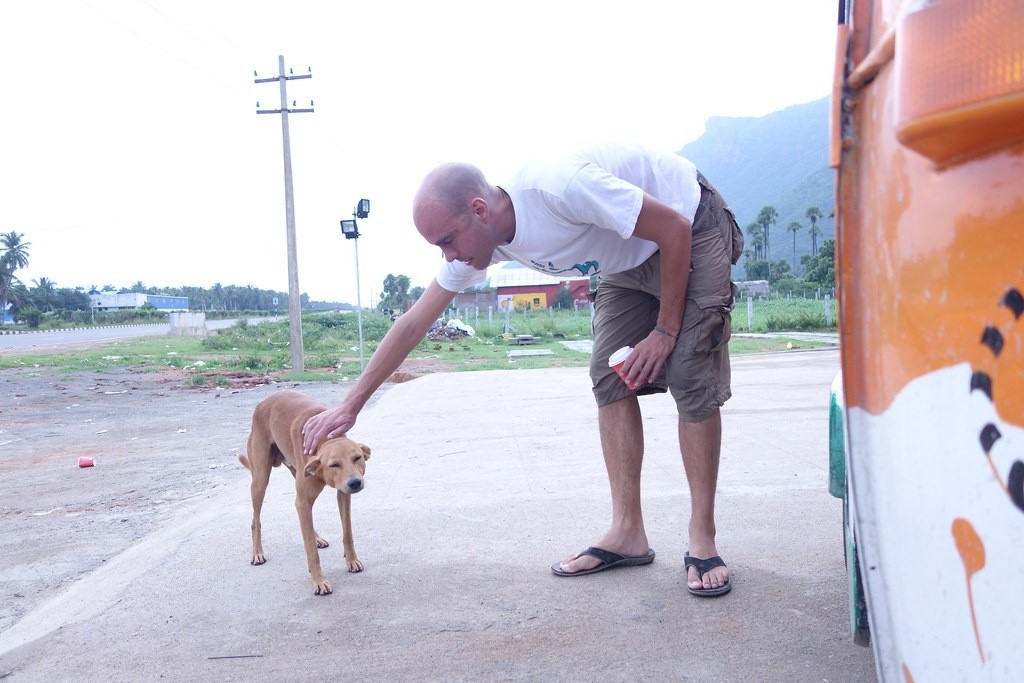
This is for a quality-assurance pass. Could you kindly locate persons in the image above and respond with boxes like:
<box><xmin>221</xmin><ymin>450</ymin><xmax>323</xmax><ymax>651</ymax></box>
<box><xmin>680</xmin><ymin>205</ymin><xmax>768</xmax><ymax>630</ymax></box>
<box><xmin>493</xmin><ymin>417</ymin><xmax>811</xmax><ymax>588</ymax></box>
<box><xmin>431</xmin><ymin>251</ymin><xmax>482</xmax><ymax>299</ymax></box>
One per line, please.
<box><xmin>301</xmin><ymin>147</ymin><xmax>745</xmax><ymax>599</ymax></box>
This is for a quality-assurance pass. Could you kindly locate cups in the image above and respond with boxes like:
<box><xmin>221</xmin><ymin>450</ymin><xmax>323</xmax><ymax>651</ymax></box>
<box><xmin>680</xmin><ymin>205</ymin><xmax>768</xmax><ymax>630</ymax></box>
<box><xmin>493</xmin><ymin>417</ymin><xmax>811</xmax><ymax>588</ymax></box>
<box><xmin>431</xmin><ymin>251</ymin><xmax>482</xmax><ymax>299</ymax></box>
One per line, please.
<box><xmin>609</xmin><ymin>345</ymin><xmax>646</xmax><ymax>390</ymax></box>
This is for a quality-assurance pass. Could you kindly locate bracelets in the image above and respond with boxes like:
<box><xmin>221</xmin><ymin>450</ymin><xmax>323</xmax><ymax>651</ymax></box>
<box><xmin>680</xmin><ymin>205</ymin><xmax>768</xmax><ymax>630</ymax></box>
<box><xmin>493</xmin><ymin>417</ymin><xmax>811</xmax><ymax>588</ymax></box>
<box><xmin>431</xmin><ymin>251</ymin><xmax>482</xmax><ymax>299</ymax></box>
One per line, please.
<box><xmin>653</xmin><ymin>324</ymin><xmax>678</xmax><ymax>341</ymax></box>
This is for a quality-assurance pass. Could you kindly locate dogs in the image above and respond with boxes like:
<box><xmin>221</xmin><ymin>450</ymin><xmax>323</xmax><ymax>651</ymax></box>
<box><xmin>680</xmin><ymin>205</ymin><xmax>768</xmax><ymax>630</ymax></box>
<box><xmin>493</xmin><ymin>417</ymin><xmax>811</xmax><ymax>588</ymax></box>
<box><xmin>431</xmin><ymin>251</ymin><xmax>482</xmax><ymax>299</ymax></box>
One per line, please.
<box><xmin>238</xmin><ymin>391</ymin><xmax>372</xmax><ymax>595</ymax></box>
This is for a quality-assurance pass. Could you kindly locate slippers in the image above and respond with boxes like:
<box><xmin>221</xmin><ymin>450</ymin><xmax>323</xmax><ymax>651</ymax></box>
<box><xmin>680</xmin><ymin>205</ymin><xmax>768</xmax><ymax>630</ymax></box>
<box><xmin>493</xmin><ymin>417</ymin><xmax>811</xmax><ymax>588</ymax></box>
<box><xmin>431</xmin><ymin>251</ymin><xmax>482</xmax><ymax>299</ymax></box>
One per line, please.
<box><xmin>684</xmin><ymin>550</ymin><xmax>734</xmax><ymax>598</ymax></box>
<box><xmin>551</xmin><ymin>544</ymin><xmax>656</xmax><ymax>576</ymax></box>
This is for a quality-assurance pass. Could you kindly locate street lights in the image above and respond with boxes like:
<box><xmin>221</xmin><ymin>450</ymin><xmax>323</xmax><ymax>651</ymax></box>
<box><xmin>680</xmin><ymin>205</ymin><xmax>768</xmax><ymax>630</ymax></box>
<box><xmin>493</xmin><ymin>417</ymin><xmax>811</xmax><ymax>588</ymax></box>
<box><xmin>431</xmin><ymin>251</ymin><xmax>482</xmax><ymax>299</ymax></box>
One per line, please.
<box><xmin>340</xmin><ymin>199</ymin><xmax>371</xmax><ymax>374</ymax></box>
